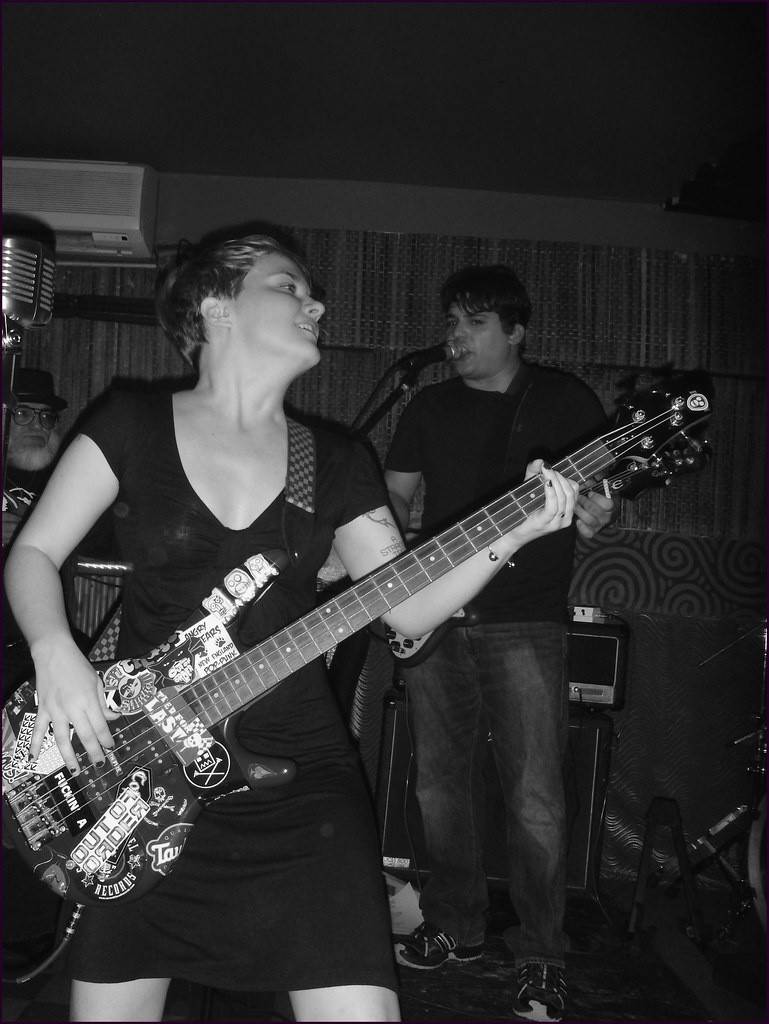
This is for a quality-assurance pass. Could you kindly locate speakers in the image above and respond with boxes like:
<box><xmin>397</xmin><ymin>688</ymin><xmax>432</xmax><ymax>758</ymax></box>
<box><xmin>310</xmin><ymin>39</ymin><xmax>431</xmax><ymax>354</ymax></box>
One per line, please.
<box><xmin>376</xmin><ymin>693</ymin><xmax>614</xmax><ymax>905</ymax></box>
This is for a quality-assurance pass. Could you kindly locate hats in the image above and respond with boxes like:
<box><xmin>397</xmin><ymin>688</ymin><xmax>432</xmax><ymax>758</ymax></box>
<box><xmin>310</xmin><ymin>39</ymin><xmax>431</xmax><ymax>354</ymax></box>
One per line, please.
<box><xmin>15</xmin><ymin>367</ymin><xmax>67</xmax><ymax>410</ymax></box>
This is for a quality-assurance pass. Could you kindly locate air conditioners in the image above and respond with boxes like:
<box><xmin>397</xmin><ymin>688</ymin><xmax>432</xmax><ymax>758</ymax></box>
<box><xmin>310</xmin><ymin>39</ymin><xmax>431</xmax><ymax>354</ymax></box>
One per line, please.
<box><xmin>2</xmin><ymin>157</ymin><xmax>160</xmax><ymax>260</ymax></box>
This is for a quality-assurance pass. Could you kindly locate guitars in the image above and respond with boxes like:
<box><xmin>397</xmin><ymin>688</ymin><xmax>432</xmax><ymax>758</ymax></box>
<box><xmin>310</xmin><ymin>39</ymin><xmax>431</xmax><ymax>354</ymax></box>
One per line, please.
<box><xmin>387</xmin><ymin>411</ymin><xmax>720</xmax><ymax>662</ymax></box>
<box><xmin>1</xmin><ymin>391</ymin><xmax>714</xmax><ymax>911</ymax></box>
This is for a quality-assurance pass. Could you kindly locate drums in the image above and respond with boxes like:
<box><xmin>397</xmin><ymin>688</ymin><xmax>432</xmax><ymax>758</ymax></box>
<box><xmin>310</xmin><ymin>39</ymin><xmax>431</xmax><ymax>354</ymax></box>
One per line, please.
<box><xmin>71</xmin><ymin>560</ymin><xmax>133</xmax><ymax>661</ymax></box>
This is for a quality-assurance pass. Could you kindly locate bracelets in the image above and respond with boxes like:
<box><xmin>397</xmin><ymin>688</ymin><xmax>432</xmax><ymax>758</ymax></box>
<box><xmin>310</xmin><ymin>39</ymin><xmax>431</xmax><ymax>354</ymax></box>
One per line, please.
<box><xmin>488</xmin><ymin>548</ymin><xmax>514</xmax><ymax>568</ymax></box>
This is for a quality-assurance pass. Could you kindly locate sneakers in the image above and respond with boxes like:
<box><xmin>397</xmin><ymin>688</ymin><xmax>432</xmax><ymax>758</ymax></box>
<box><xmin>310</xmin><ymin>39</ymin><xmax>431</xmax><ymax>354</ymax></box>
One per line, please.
<box><xmin>512</xmin><ymin>958</ymin><xmax>566</xmax><ymax>1023</ymax></box>
<box><xmin>392</xmin><ymin>921</ymin><xmax>486</xmax><ymax>970</ymax></box>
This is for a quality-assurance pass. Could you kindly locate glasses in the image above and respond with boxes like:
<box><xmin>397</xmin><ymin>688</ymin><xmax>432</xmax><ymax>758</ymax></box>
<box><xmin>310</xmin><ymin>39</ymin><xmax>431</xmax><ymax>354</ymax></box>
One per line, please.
<box><xmin>12</xmin><ymin>407</ymin><xmax>60</xmax><ymax>430</ymax></box>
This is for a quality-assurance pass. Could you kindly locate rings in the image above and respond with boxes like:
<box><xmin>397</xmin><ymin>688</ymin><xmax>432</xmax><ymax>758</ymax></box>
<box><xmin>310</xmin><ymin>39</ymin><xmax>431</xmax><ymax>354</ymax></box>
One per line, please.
<box><xmin>556</xmin><ymin>511</ymin><xmax>566</xmax><ymax>517</ymax></box>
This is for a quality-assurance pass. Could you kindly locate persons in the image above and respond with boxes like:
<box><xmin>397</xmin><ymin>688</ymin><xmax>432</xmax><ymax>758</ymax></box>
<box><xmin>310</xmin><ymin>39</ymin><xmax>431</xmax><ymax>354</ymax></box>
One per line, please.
<box><xmin>2</xmin><ymin>233</ymin><xmax>578</xmax><ymax>1022</ymax></box>
<box><xmin>383</xmin><ymin>268</ymin><xmax>614</xmax><ymax>1022</ymax></box>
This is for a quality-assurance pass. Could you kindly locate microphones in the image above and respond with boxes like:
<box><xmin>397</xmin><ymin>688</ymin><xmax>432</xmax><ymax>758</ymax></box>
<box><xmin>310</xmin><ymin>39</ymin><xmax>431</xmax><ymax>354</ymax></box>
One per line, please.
<box><xmin>1</xmin><ymin>236</ymin><xmax>56</xmax><ymax>352</ymax></box>
<box><xmin>397</xmin><ymin>340</ymin><xmax>461</xmax><ymax>370</ymax></box>
